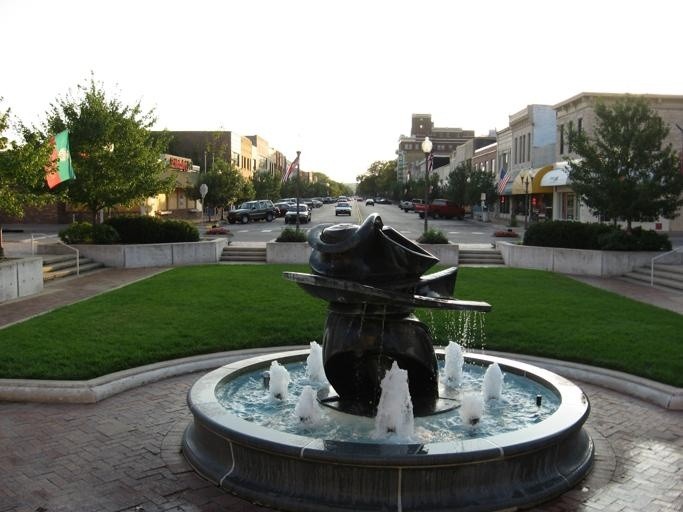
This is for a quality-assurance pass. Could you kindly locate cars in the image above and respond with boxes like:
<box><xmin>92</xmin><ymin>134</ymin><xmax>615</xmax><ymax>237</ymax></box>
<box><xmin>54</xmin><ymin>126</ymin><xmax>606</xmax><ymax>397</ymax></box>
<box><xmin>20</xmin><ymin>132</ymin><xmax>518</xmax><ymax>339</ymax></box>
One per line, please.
<box><xmin>380</xmin><ymin>199</ymin><xmax>388</xmax><ymax>202</ymax></box>
<box><xmin>275</xmin><ymin>195</ymin><xmax>374</xmax><ymax>224</ymax></box>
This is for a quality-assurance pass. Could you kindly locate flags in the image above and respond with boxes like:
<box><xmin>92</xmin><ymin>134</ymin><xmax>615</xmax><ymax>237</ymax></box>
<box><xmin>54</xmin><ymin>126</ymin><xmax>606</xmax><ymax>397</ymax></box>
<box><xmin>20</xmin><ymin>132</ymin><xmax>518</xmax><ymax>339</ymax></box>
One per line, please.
<box><xmin>280</xmin><ymin>155</ymin><xmax>299</xmax><ymax>183</ymax></box>
<box><xmin>495</xmin><ymin>167</ymin><xmax>510</xmax><ymax>196</ymax></box>
<box><xmin>39</xmin><ymin>128</ymin><xmax>77</xmax><ymax>191</ymax></box>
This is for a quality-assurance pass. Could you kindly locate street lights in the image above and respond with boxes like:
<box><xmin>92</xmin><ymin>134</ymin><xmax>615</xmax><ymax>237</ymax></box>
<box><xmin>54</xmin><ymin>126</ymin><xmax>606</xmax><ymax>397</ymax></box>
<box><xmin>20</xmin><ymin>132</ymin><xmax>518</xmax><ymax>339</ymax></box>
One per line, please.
<box><xmin>519</xmin><ymin>171</ymin><xmax>536</xmax><ymax>231</ymax></box>
<box><xmin>422</xmin><ymin>136</ymin><xmax>433</xmax><ymax>232</ymax></box>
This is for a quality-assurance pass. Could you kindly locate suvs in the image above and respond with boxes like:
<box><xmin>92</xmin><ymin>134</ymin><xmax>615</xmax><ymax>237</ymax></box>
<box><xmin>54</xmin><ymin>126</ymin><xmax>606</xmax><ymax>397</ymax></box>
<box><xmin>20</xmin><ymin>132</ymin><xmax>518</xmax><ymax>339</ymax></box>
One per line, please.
<box><xmin>226</xmin><ymin>199</ymin><xmax>275</xmax><ymax>224</ymax></box>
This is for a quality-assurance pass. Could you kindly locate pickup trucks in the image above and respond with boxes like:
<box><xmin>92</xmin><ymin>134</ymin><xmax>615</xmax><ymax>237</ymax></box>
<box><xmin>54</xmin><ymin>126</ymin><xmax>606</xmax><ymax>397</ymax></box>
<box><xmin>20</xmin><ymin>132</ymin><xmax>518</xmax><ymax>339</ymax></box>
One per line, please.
<box><xmin>401</xmin><ymin>199</ymin><xmax>425</xmax><ymax>213</ymax></box>
<box><xmin>415</xmin><ymin>199</ymin><xmax>466</xmax><ymax>220</ymax></box>
